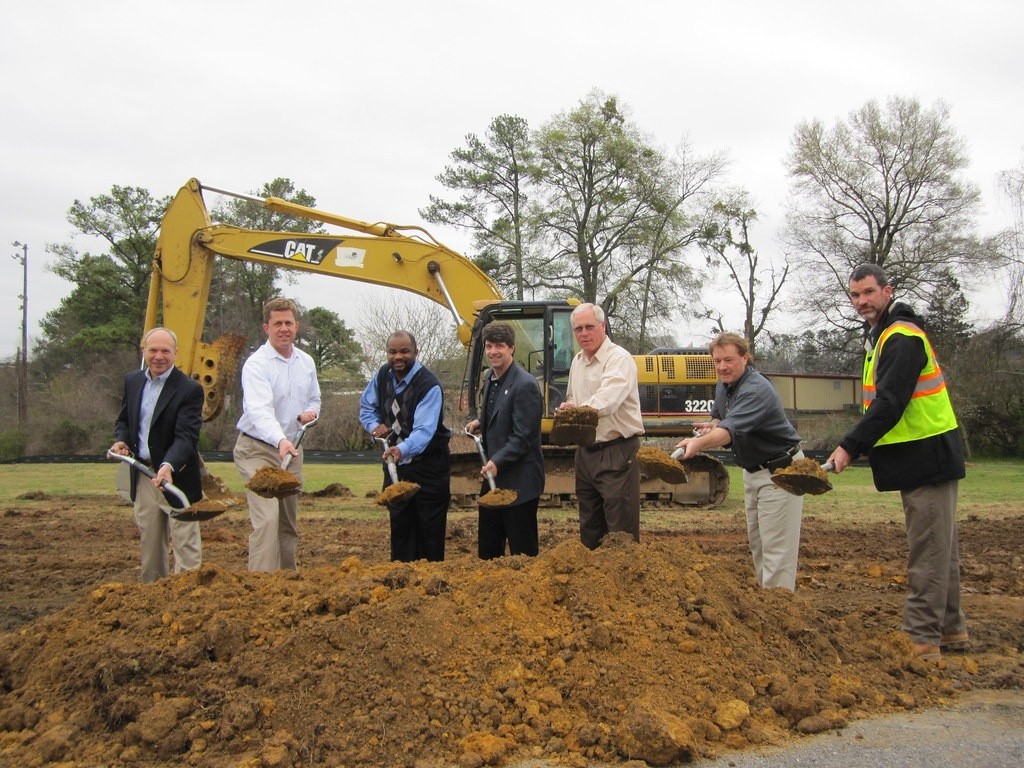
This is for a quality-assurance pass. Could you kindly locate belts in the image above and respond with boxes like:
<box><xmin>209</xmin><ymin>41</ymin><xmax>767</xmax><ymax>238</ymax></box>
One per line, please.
<box><xmin>587</xmin><ymin>435</ymin><xmax>625</xmax><ymax>453</ymax></box>
<box><xmin>136</xmin><ymin>459</ymin><xmax>154</xmax><ymax>467</ymax></box>
<box><xmin>243</xmin><ymin>432</ymin><xmax>273</xmax><ymax>447</ymax></box>
<box><xmin>745</xmin><ymin>443</ymin><xmax>803</xmax><ymax>473</ymax></box>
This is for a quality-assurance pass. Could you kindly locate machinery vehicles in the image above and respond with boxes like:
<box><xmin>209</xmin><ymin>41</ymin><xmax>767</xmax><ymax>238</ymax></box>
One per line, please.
<box><xmin>141</xmin><ymin>181</ymin><xmax>752</xmax><ymax>512</ymax></box>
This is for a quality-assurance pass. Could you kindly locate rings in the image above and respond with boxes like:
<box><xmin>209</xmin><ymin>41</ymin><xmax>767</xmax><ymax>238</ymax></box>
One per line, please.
<box><xmin>373</xmin><ymin>432</ymin><xmax>377</xmax><ymax>435</ymax></box>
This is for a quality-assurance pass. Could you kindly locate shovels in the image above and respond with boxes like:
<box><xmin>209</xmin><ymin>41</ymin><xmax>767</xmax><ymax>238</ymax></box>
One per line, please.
<box><xmin>638</xmin><ymin>426</ymin><xmax>711</xmax><ymax>484</ymax></box>
<box><xmin>464</xmin><ymin>426</ymin><xmax>519</xmax><ymax>508</ymax></box>
<box><xmin>771</xmin><ymin>461</ymin><xmax>834</xmax><ymax>497</ymax></box>
<box><xmin>550</xmin><ymin>409</ymin><xmax>600</xmax><ymax>448</ymax></box>
<box><xmin>372</xmin><ymin>426</ymin><xmax>420</xmax><ymax>505</ymax></box>
<box><xmin>251</xmin><ymin>413</ymin><xmax>320</xmax><ymax>498</ymax></box>
<box><xmin>106</xmin><ymin>448</ymin><xmax>226</xmax><ymax>521</ymax></box>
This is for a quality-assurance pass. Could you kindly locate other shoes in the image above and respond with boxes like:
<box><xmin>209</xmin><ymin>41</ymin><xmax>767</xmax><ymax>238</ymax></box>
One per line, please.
<box><xmin>940</xmin><ymin>631</ymin><xmax>971</xmax><ymax>650</ymax></box>
<box><xmin>908</xmin><ymin>638</ymin><xmax>942</xmax><ymax>659</ymax></box>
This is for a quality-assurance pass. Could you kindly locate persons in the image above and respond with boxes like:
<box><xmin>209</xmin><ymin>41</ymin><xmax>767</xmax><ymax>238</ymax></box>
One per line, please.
<box><xmin>359</xmin><ymin>329</ymin><xmax>454</xmax><ymax>564</ymax></box>
<box><xmin>233</xmin><ymin>297</ymin><xmax>322</xmax><ymax>573</ymax></box>
<box><xmin>107</xmin><ymin>327</ymin><xmax>205</xmax><ymax>586</ymax></box>
<box><xmin>826</xmin><ymin>264</ymin><xmax>966</xmax><ymax>659</ymax></box>
<box><xmin>464</xmin><ymin>321</ymin><xmax>545</xmax><ymax>560</ymax></box>
<box><xmin>560</xmin><ymin>302</ymin><xmax>645</xmax><ymax>551</ymax></box>
<box><xmin>676</xmin><ymin>332</ymin><xmax>805</xmax><ymax>592</ymax></box>
